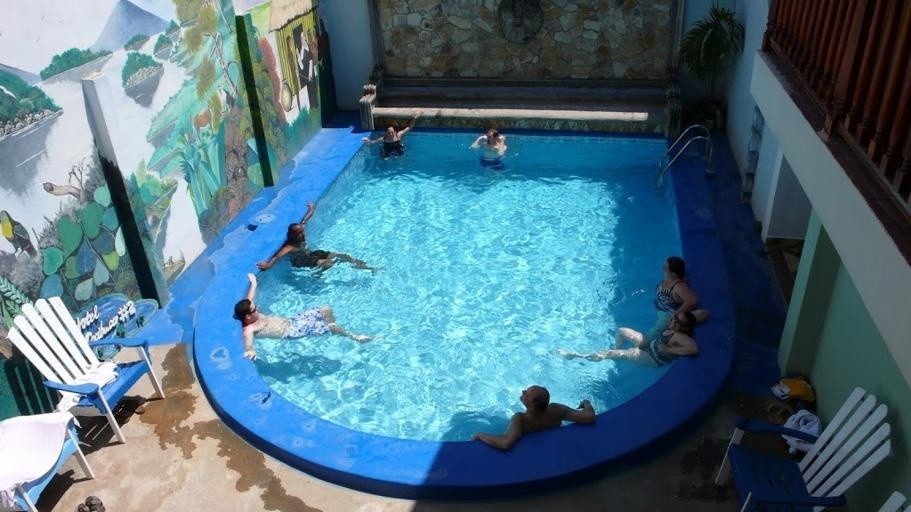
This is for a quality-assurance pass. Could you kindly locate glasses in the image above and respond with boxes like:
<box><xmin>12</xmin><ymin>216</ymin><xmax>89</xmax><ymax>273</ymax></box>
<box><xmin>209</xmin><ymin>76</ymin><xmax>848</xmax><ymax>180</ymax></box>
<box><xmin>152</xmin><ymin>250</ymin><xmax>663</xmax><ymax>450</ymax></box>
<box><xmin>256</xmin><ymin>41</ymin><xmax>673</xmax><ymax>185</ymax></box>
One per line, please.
<box><xmin>244</xmin><ymin>305</ymin><xmax>256</xmax><ymax>316</ymax></box>
<box><xmin>674</xmin><ymin>314</ymin><xmax>687</xmax><ymax>327</ymax></box>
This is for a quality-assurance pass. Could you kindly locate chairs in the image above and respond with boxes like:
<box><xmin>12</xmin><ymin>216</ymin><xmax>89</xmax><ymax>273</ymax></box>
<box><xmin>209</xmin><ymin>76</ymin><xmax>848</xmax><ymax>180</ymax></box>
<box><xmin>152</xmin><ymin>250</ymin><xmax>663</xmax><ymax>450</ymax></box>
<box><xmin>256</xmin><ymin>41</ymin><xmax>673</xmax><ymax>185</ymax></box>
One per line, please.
<box><xmin>713</xmin><ymin>387</ymin><xmax>895</xmax><ymax>510</ymax></box>
<box><xmin>0</xmin><ymin>297</ymin><xmax>168</xmax><ymax>512</ymax></box>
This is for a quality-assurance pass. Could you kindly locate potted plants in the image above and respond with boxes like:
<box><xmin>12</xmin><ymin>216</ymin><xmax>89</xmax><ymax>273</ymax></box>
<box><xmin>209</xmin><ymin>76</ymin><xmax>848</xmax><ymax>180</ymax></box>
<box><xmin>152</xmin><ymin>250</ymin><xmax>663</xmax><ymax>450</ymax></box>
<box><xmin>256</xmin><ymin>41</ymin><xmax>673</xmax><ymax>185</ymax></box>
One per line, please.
<box><xmin>677</xmin><ymin>3</ymin><xmax>746</xmax><ymax>134</ymax></box>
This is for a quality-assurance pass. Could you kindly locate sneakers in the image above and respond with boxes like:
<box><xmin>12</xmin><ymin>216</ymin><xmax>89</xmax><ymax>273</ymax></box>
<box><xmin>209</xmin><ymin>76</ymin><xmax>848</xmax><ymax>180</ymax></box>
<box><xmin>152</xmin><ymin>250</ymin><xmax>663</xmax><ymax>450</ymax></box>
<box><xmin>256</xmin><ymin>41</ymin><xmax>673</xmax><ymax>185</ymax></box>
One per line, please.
<box><xmin>125</xmin><ymin>401</ymin><xmax>145</xmax><ymax>414</ymax></box>
<box><xmin>115</xmin><ymin>414</ymin><xmax>130</xmax><ymax>424</ymax></box>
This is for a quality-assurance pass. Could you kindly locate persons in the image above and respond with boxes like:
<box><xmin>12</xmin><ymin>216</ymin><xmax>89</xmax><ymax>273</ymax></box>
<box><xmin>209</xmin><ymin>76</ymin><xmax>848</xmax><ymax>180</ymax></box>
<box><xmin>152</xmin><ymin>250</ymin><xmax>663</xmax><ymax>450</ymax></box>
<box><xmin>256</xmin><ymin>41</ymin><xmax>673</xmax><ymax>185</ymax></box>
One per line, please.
<box><xmin>362</xmin><ymin>113</ymin><xmax>421</xmax><ymax>159</ymax></box>
<box><xmin>255</xmin><ymin>203</ymin><xmax>376</xmax><ymax>277</ymax></box>
<box><xmin>1</xmin><ymin>108</ymin><xmax>57</xmax><ymax>139</ymax></box>
<box><xmin>557</xmin><ymin>309</ymin><xmax>709</xmax><ymax>368</ymax></box>
<box><xmin>234</xmin><ymin>271</ymin><xmax>374</xmax><ymax>362</ymax></box>
<box><xmin>286</xmin><ymin>19</ymin><xmax>336</xmax><ymax>117</ymax></box>
<box><xmin>471</xmin><ymin>129</ymin><xmax>508</xmax><ymax>172</ymax></box>
<box><xmin>472</xmin><ymin>385</ymin><xmax>596</xmax><ymax>450</ymax></box>
<box><xmin>653</xmin><ymin>256</ymin><xmax>700</xmax><ymax>333</ymax></box>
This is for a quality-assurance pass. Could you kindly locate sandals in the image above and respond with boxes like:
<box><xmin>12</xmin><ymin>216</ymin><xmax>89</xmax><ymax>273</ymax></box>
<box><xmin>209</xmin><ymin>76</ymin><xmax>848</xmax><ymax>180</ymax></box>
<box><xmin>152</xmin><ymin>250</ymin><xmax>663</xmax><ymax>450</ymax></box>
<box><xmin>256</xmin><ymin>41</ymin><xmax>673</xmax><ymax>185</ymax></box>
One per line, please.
<box><xmin>78</xmin><ymin>496</ymin><xmax>105</xmax><ymax>512</ymax></box>
